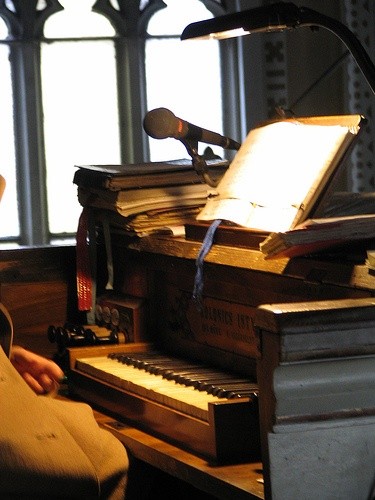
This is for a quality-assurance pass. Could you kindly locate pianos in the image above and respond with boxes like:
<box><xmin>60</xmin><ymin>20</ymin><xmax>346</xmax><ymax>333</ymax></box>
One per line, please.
<box><xmin>65</xmin><ymin>341</ymin><xmax>263</xmax><ymax>466</ymax></box>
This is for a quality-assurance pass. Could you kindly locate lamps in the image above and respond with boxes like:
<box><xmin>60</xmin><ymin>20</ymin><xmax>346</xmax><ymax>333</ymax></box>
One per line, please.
<box><xmin>181</xmin><ymin>2</ymin><xmax>375</xmax><ymax>97</ymax></box>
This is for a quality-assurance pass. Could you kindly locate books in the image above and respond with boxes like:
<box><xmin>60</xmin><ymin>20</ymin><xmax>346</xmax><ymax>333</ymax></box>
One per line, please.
<box><xmin>73</xmin><ymin>158</ymin><xmax>230</xmax><ymax>240</ymax></box>
<box><xmin>197</xmin><ymin>121</ymin><xmax>357</xmax><ymax>235</ymax></box>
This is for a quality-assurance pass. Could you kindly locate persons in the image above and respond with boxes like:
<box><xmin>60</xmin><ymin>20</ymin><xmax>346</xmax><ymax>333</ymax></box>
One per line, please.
<box><xmin>0</xmin><ymin>303</ymin><xmax>132</xmax><ymax>500</ymax></box>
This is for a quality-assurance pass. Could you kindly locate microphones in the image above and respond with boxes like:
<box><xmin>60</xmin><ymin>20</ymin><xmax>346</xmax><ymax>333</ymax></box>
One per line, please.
<box><xmin>143</xmin><ymin>107</ymin><xmax>241</xmax><ymax>151</ymax></box>
